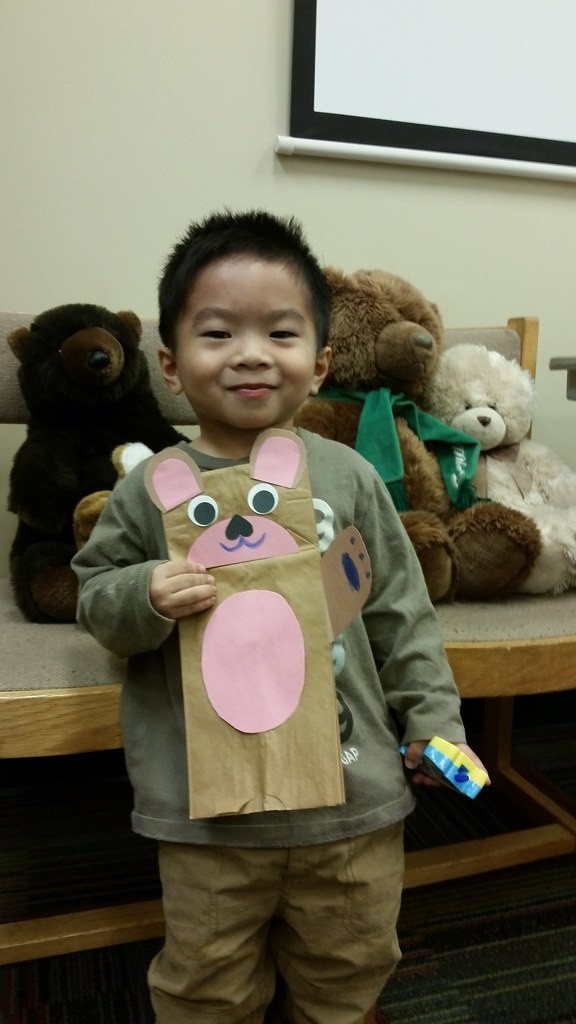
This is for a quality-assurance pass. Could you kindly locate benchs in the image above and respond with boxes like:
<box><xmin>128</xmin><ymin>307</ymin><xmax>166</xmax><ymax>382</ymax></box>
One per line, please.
<box><xmin>0</xmin><ymin>314</ymin><xmax>576</xmax><ymax>967</ymax></box>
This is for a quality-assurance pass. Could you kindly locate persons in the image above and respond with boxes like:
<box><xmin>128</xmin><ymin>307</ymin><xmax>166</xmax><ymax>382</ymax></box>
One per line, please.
<box><xmin>69</xmin><ymin>210</ymin><xmax>491</xmax><ymax>1024</ymax></box>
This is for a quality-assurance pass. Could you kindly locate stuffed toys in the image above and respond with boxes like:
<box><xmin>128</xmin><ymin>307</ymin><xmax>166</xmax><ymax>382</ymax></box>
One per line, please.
<box><xmin>6</xmin><ymin>303</ymin><xmax>195</xmax><ymax>626</ymax></box>
<box><xmin>422</xmin><ymin>344</ymin><xmax>576</xmax><ymax>596</ymax></box>
<box><xmin>292</xmin><ymin>266</ymin><xmax>542</xmax><ymax>603</ymax></box>
